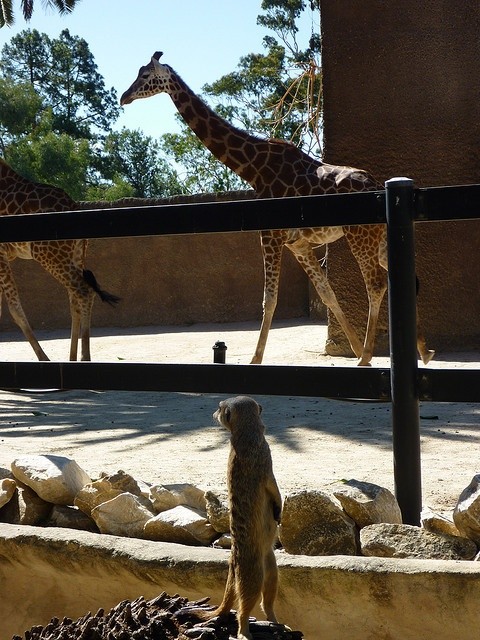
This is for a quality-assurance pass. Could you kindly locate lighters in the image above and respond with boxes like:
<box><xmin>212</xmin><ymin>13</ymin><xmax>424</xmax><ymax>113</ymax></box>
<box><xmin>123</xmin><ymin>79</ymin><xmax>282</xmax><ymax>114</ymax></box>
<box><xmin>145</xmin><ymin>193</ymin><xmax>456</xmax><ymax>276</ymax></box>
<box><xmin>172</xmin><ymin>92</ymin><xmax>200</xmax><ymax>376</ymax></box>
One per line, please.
<box><xmin>119</xmin><ymin>49</ymin><xmax>437</xmax><ymax>370</ymax></box>
<box><xmin>0</xmin><ymin>157</ymin><xmax>123</xmax><ymax>362</ymax></box>
